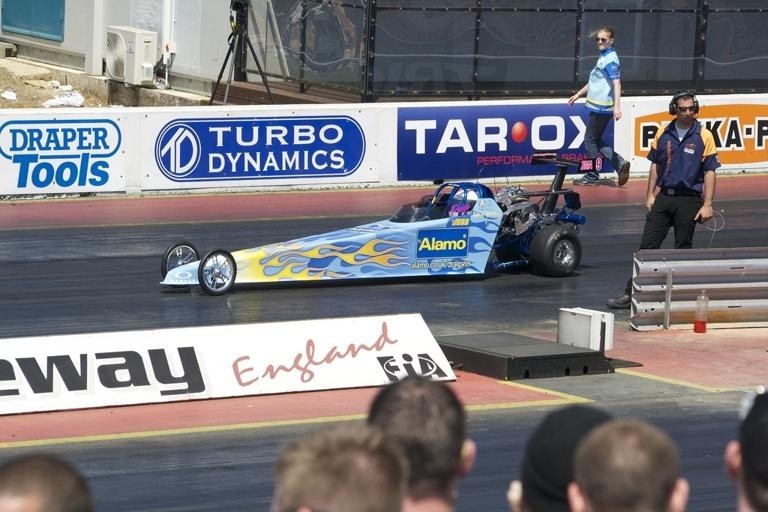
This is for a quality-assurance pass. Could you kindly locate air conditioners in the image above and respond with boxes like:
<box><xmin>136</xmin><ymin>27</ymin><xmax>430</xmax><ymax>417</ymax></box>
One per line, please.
<box><xmin>104</xmin><ymin>25</ymin><xmax>156</xmax><ymax>88</ymax></box>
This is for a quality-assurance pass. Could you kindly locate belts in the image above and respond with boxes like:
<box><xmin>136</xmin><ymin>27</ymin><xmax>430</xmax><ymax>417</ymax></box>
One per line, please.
<box><xmin>660</xmin><ymin>188</ymin><xmax>701</xmax><ymax>197</ymax></box>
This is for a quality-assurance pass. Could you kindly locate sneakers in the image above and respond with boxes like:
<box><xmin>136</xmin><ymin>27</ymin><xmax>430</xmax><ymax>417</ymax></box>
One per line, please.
<box><xmin>606</xmin><ymin>294</ymin><xmax>632</xmax><ymax>309</ymax></box>
<box><xmin>618</xmin><ymin>161</ymin><xmax>630</xmax><ymax>186</ymax></box>
<box><xmin>573</xmin><ymin>179</ymin><xmax>599</xmax><ymax>187</ymax></box>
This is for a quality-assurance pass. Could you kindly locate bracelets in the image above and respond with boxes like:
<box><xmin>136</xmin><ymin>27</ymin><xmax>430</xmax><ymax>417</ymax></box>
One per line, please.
<box><xmin>576</xmin><ymin>93</ymin><xmax>581</xmax><ymax>98</ymax></box>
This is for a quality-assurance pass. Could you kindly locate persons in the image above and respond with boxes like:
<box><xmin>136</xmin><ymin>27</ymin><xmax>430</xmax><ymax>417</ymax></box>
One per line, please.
<box><xmin>720</xmin><ymin>387</ymin><xmax>767</xmax><ymax>511</ymax></box>
<box><xmin>566</xmin><ymin>24</ymin><xmax>630</xmax><ymax>187</ymax></box>
<box><xmin>265</xmin><ymin>419</ymin><xmax>409</xmax><ymax>512</ymax></box>
<box><xmin>0</xmin><ymin>449</ymin><xmax>93</xmax><ymax>512</ymax></box>
<box><xmin>565</xmin><ymin>416</ymin><xmax>691</xmax><ymax>512</ymax></box>
<box><xmin>503</xmin><ymin>403</ymin><xmax>615</xmax><ymax>512</ymax></box>
<box><xmin>447</xmin><ymin>187</ymin><xmax>478</xmax><ymax>218</ymax></box>
<box><xmin>604</xmin><ymin>87</ymin><xmax>720</xmax><ymax>311</ymax></box>
<box><xmin>367</xmin><ymin>371</ymin><xmax>479</xmax><ymax>512</ymax></box>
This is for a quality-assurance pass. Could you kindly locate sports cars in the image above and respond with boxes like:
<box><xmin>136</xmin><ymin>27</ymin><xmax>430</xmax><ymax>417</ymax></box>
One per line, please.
<box><xmin>158</xmin><ymin>149</ymin><xmax>614</xmax><ymax>299</ymax></box>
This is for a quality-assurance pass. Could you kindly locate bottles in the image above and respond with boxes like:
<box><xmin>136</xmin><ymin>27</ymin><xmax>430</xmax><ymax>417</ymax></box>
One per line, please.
<box><xmin>694</xmin><ymin>288</ymin><xmax>709</xmax><ymax>333</ymax></box>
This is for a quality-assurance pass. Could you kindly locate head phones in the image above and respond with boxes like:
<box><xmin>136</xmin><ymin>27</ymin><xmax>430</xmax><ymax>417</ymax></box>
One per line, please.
<box><xmin>668</xmin><ymin>91</ymin><xmax>700</xmax><ymax>115</ymax></box>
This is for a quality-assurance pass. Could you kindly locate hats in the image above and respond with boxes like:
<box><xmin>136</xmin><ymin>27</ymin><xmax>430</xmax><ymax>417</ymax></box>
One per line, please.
<box><xmin>521</xmin><ymin>403</ymin><xmax>612</xmax><ymax>510</ymax></box>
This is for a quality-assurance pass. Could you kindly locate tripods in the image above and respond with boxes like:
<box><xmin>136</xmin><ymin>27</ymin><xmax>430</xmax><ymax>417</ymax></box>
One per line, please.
<box><xmin>208</xmin><ymin>24</ymin><xmax>274</xmax><ymax>105</ymax></box>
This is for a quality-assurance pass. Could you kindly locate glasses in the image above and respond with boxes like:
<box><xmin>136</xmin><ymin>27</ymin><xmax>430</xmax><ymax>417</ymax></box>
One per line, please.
<box><xmin>676</xmin><ymin>105</ymin><xmax>695</xmax><ymax>112</ymax></box>
<box><xmin>596</xmin><ymin>37</ymin><xmax>606</xmax><ymax>43</ymax></box>
